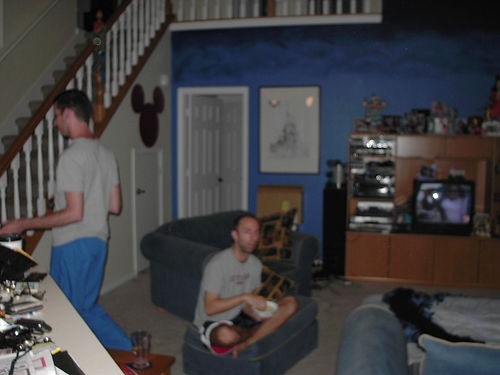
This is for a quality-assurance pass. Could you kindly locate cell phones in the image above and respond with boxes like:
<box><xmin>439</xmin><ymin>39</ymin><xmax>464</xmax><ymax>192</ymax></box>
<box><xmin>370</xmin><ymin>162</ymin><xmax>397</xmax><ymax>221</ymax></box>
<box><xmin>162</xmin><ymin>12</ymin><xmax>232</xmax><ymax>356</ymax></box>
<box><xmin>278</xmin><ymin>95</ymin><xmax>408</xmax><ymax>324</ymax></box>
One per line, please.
<box><xmin>23</xmin><ymin>272</ymin><xmax>47</xmax><ymax>283</ymax></box>
<box><xmin>15</xmin><ymin>318</ymin><xmax>52</xmax><ymax>332</ymax></box>
<box><xmin>10</xmin><ymin>302</ymin><xmax>43</xmax><ymax>314</ymax></box>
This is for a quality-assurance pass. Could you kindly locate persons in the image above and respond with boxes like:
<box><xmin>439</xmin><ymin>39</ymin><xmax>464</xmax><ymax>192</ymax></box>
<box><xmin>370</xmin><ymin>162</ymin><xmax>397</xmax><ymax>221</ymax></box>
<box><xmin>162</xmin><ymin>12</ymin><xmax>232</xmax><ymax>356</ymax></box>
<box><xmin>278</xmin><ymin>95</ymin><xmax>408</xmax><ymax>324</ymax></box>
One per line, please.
<box><xmin>0</xmin><ymin>90</ymin><xmax>137</xmax><ymax>352</ymax></box>
<box><xmin>191</xmin><ymin>214</ymin><xmax>299</xmax><ymax>360</ymax></box>
<box><xmin>422</xmin><ymin>184</ymin><xmax>470</xmax><ymax>223</ymax></box>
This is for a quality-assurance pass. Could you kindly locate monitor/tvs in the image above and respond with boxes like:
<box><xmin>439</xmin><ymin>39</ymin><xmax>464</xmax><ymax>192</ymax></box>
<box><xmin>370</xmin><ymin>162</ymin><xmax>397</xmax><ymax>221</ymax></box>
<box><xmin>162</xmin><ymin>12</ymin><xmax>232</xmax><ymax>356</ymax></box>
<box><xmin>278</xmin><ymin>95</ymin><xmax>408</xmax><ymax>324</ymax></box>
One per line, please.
<box><xmin>411</xmin><ymin>177</ymin><xmax>475</xmax><ymax>235</ymax></box>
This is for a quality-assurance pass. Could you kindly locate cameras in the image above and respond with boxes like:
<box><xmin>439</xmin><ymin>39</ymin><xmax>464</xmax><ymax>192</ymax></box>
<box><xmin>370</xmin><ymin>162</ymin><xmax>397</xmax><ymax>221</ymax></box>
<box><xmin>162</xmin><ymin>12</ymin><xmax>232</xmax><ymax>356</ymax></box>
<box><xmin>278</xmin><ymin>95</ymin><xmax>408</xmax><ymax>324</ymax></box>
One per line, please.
<box><xmin>0</xmin><ymin>326</ymin><xmax>35</xmax><ymax>351</ymax></box>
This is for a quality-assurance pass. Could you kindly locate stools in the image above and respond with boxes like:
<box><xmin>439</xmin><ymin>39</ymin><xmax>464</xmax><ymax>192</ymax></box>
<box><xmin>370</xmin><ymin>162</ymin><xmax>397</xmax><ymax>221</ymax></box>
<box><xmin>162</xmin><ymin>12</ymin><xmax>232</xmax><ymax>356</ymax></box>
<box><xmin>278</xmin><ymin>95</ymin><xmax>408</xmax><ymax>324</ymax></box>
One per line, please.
<box><xmin>182</xmin><ymin>296</ymin><xmax>319</xmax><ymax>375</ymax></box>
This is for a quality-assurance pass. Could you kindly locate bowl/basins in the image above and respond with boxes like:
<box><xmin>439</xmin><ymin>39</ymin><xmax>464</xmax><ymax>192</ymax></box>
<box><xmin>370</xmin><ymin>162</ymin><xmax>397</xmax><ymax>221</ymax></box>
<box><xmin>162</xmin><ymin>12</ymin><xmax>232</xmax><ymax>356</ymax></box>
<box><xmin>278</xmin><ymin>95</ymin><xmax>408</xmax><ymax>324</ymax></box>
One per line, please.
<box><xmin>252</xmin><ymin>299</ymin><xmax>279</xmax><ymax>318</ymax></box>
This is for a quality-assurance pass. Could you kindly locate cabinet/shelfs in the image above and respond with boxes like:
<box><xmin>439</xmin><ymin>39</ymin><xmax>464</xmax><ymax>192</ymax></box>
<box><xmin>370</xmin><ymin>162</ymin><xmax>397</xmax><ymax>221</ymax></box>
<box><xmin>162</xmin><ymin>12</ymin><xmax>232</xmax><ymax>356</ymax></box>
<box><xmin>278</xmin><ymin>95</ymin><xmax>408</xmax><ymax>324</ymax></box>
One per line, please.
<box><xmin>345</xmin><ymin>132</ymin><xmax>500</xmax><ymax>298</ymax></box>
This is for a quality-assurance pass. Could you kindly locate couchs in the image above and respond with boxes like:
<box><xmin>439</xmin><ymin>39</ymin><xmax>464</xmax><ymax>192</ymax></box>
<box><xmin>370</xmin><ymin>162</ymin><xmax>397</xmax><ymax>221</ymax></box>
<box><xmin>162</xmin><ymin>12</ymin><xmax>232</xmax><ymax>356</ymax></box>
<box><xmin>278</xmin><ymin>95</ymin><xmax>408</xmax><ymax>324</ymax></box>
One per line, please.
<box><xmin>335</xmin><ymin>306</ymin><xmax>500</xmax><ymax>375</ymax></box>
<box><xmin>140</xmin><ymin>211</ymin><xmax>319</xmax><ymax>323</ymax></box>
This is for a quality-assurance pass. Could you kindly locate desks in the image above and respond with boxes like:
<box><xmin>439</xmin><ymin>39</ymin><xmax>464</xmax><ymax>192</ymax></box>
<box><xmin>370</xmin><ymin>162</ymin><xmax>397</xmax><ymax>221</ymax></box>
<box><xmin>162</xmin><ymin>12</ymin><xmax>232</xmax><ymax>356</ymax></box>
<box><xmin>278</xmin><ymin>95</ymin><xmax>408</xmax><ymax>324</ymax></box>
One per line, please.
<box><xmin>107</xmin><ymin>346</ymin><xmax>176</xmax><ymax>375</ymax></box>
<box><xmin>31</xmin><ymin>266</ymin><xmax>126</xmax><ymax>375</ymax></box>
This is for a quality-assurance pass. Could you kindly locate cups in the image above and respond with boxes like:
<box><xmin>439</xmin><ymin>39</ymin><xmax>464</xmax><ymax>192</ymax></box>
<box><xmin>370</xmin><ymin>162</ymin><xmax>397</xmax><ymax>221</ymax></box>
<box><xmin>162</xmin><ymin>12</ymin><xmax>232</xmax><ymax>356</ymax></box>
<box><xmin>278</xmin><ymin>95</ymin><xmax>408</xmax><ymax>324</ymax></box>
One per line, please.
<box><xmin>131</xmin><ymin>330</ymin><xmax>151</xmax><ymax>369</ymax></box>
